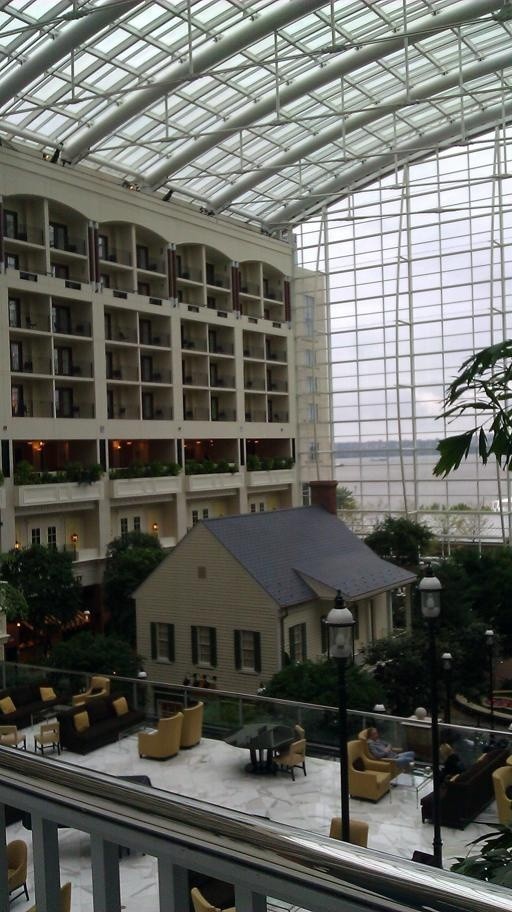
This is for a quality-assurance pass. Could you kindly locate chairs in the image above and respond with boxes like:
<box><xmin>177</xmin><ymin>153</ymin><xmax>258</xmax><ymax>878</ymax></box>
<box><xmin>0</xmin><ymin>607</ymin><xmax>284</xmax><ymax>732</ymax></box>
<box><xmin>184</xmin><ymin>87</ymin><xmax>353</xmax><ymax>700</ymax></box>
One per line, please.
<box><xmin>0</xmin><ymin>725</ymin><xmax>26</xmax><ymax>751</ymax></box>
<box><xmin>139</xmin><ymin>701</ymin><xmax>204</xmax><ymax>759</ymax></box>
<box><xmin>343</xmin><ymin>728</ymin><xmax>405</xmax><ymax>804</ymax></box>
<box><xmin>72</xmin><ymin>676</ymin><xmax>111</xmax><ymax>708</ymax></box>
<box><xmin>490</xmin><ymin>753</ymin><xmax>512</xmax><ymax>830</ymax></box>
<box><xmin>272</xmin><ymin>724</ymin><xmax>308</xmax><ymax>783</ymax></box>
<box><xmin>7</xmin><ymin>838</ymin><xmax>71</xmax><ymax>911</ymax></box>
<box><xmin>34</xmin><ymin>722</ymin><xmax>60</xmax><ymax>756</ymax></box>
<box><xmin>331</xmin><ymin>816</ymin><xmax>369</xmax><ymax>851</ymax></box>
<box><xmin>190</xmin><ymin>885</ymin><xmax>237</xmax><ymax>912</ymax></box>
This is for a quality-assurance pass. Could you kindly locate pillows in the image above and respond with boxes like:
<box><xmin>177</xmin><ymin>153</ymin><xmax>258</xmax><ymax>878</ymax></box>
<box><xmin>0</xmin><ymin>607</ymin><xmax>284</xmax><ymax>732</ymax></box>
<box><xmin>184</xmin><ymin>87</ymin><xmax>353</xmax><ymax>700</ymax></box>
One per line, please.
<box><xmin>0</xmin><ymin>695</ymin><xmax>17</xmax><ymax>715</ymax></box>
<box><xmin>111</xmin><ymin>696</ymin><xmax>129</xmax><ymax>716</ymax></box>
<box><xmin>39</xmin><ymin>686</ymin><xmax>56</xmax><ymax>702</ymax></box>
<box><xmin>73</xmin><ymin>710</ymin><xmax>90</xmax><ymax>731</ymax></box>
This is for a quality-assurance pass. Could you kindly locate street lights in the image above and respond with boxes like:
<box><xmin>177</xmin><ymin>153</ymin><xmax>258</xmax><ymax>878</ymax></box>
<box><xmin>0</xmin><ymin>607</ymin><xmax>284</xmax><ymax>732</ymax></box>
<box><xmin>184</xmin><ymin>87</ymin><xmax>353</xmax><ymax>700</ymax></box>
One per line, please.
<box><xmin>441</xmin><ymin>653</ymin><xmax>453</xmax><ymax>723</ymax></box>
<box><xmin>322</xmin><ymin>590</ymin><xmax>356</xmax><ymax>843</ymax></box>
<box><xmin>484</xmin><ymin>629</ymin><xmax>495</xmax><ymax>745</ymax></box>
<box><xmin>419</xmin><ymin>568</ymin><xmax>443</xmax><ymax>868</ymax></box>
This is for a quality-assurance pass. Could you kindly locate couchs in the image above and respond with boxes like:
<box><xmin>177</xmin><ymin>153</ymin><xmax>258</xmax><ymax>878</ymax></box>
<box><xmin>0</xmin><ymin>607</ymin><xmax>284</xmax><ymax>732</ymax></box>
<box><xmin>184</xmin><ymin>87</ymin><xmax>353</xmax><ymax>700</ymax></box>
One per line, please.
<box><xmin>57</xmin><ymin>692</ymin><xmax>147</xmax><ymax>757</ymax></box>
<box><xmin>0</xmin><ymin>682</ymin><xmax>69</xmax><ymax>726</ymax></box>
<box><xmin>420</xmin><ymin>736</ymin><xmax>512</xmax><ymax>833</ymax></box>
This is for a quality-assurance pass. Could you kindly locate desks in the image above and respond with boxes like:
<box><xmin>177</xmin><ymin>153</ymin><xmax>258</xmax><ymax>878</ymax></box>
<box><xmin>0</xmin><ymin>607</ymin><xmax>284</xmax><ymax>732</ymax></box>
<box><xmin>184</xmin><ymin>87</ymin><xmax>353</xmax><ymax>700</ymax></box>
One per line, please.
<box><xmin>223</xmin><ymin>723</ymin><xmax>301</xmax><ymax>775</ymax></box>
<box><xmin>21</xmin><ymin>776</ymin><xmax>155</xmax><ymax>860</ymax></box>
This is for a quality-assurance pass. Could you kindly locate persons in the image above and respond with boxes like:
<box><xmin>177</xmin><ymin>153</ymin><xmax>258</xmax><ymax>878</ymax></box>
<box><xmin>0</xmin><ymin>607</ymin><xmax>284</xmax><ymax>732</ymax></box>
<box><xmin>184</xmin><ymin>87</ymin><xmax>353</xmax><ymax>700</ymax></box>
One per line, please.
<box><xmin>438</xmin><ymin>739</ymin><xmax>461</xmax><ymax>779</ymax></box>
<box><xmin>367</xmin><ymin>725</ymin><xmax>415</xmax><ymax>766</ymax></box>
